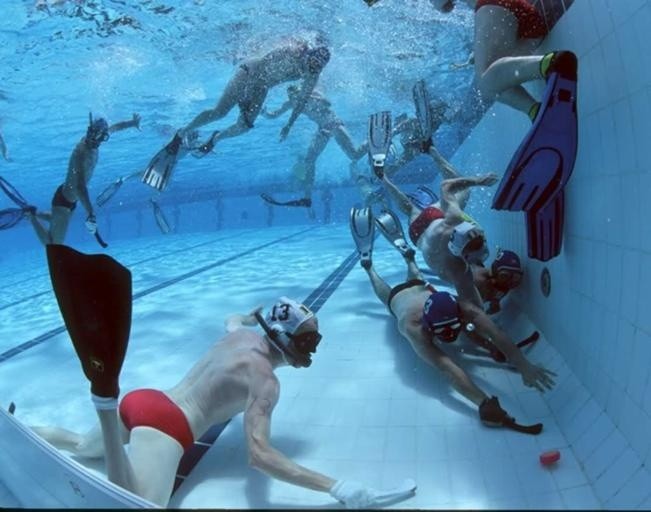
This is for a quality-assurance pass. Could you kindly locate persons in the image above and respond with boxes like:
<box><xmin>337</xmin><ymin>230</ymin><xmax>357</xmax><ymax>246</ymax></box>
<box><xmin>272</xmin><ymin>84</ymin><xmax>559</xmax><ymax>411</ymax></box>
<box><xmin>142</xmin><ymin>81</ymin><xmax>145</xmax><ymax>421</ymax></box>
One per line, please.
<box><xmin>7</xmin><ymin>294</ymin><xmax>381</xmax><ymax>512</ymax></box>
<box><xmin>255</xmin><ymin>0</ymin><xmax>579</xmax><ymax>428</ymax></box>
<box><xmin>15</xmin><ymin>112</ymin><xmax>142</xmax><ymax>249</ymax></box>
<box><xmin>171</xmin><ymin>39</ymin><xmax>331</xmax><ymax>159</ymax></box>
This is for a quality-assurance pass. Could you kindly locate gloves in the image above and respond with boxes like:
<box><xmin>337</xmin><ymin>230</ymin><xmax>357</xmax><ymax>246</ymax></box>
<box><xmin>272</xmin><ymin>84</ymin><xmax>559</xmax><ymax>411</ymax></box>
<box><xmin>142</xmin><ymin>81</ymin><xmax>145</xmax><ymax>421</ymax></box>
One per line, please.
<box><xmin>329</xmin><ymin>479</ymin><xmax>376</xmax><ymax>509</ymax></box>
<box><xmin>479</xmin><ymin>395</ymin><xmax>516</xmax><ymax>426</ymax></box>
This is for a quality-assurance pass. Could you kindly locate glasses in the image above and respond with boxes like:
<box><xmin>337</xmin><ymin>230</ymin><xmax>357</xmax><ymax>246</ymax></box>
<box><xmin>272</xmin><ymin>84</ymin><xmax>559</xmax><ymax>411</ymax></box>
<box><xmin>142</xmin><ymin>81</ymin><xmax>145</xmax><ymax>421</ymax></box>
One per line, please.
<box><xmin>429</xmin><ymin>317</ymin><xmax>461</xmax><ymax>335</ymax></box>
<box><xmin>495</xmin><ymin>268</ymin><xmax>523</xmax><ymax>282</ymax></box>
<box><xmin>285</xmin><ymin>332</ymin><xmax>322</xmax><ymax>353</ymax></box>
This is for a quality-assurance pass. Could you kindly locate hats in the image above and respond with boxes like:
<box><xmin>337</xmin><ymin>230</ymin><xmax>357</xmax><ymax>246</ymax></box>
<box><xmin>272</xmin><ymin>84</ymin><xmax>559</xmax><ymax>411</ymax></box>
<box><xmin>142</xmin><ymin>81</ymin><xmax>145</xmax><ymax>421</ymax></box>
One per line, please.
<box><xmin>422</xmin><ymin>291</ymin><xmax>459</xmax><ymax>329</ymax></box>
<box><xmin>265</xmin><ymin>296</ymin><xmax>314</xmax><ymax>353</ymax></box>
<box><xmin>491</xmin><ymin>250</ymin><xmax>523</xmax><ymax>278</ymax></box>
<box><xmin>447</xmin><ymin>221</ymin><xmax>484</xmax><ymax>258</ymax></box>
